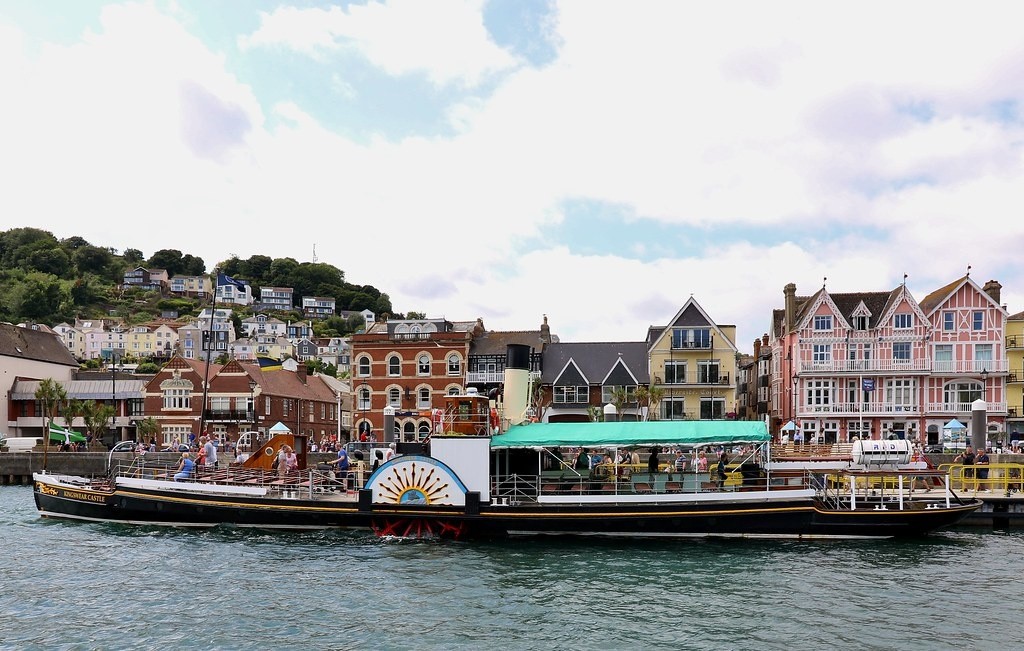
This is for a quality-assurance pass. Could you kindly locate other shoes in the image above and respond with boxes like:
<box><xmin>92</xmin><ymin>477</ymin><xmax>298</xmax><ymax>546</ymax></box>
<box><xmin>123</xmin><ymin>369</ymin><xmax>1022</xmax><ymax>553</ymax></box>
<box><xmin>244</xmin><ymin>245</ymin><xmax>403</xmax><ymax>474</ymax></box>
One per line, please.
<box><xmin>924</xmin><ymin>489</ymin><xmax>931</xmax><ymax>493</ymax></box>
<box><xmin>908</xmin><ymin>489</ymin><xmax>915</xmax><ymax>492</ymax></box>
<box><xmin>960</xmin><ymin>488</ymin><xmax>968</xmax><ymax>492</ymax></box>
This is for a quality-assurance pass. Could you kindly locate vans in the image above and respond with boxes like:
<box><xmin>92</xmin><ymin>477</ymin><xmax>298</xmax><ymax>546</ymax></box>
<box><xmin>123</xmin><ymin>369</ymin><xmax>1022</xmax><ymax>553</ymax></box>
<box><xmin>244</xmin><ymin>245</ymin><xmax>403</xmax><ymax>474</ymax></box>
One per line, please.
<box><xmin>0</xmin><ymin>435</ymin><xmax>43</xmax><ymax>454</ymax></box>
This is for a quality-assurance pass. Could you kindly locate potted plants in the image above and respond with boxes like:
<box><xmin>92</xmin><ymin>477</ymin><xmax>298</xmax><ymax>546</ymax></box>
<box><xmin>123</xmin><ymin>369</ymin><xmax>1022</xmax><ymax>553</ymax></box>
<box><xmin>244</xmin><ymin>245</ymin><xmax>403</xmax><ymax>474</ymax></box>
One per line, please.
<box><xmin>138</xmin><ymin>416</ymin><xmax>159</xmax><ymax>445</ymax></box>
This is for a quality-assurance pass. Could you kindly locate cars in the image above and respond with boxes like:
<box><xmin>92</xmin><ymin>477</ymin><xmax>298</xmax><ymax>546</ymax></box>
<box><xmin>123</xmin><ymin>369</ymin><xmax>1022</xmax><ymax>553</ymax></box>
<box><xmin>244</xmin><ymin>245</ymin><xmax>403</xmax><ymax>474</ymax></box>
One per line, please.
<box><xmin>160</xmin><ymin>443</ymin><xmax>192</xmax><ymax>452</ymax></box>
<box><xmin>923</xmin><ymin>444</ymin><xmax>950</xmax><ymax>454</ymax></box>
<box><xmin>113</xmin><ymin>442</ymin><xmax>149</xmax><ymax>451</ymax></box>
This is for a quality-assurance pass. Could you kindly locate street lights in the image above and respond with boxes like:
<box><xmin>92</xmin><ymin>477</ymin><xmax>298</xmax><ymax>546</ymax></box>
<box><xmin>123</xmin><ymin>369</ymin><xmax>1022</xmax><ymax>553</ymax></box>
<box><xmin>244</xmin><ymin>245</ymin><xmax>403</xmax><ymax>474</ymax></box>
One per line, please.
<box><xmin>360</xmin><ymin>378</ymin><xmax>369</xmax><ymax>430</ymax></box>
<box><xmin>979</xmin><ymin>368</ymin><xmax>989</xmax><ymax>401</ymax></box>
<box><xmin>102</xmin><ymin>351</ymin><xmax>125</xmax><ymax>442</ymax></box>
<box><xmin>247</xmin><ymin>379</ymin><xmax>257</xmax><ymax>452</ymax></box>
<box><xmin>791</xmin><ymin>372</ymin><xmax>800</xmax><ymax>434</ymax></box>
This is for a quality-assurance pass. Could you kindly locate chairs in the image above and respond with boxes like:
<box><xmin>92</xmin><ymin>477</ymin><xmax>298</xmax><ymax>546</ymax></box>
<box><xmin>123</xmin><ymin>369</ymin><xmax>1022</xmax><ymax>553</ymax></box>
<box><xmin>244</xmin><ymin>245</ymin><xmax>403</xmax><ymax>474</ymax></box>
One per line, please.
<box><xmin>722</xmin><ymin>376</ymin><xmax>728</xmax><ymax>384</ymax></box>
<box><xmin>654</xmin><ymin>372</ymin><xmax>661</xmax><ymax>384</ymax></box>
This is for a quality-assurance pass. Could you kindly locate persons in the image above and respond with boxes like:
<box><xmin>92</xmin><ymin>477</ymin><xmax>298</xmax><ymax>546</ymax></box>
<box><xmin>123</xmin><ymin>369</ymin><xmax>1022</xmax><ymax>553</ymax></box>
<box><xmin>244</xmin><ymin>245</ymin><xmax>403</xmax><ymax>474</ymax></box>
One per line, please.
<box><xmin>234</xmin><ymin>449</ymin><xmax>245</xmax><ymax>463</ymax></box>
<box><xmin>194</xmin><ymin>434</ymin><xmax>219</xmax><ymax>469</ymax></box>
<box><xmin>906</xmin><ymin>428</ymin><xmax>931</xmax><ymax>493</ymax></box>
<box><xmin>57</xmin><ymin>440</ymin><xmax>70</xmax><ymax>452</ymax></box>
<box><xmin>780</xmin><ymin>430</ymin><xmax>816</xmax><ymax>452</ymax></box>
<box><xmin>174</xmin><ymin>452</ymin><xmax>193</xmax><ymax>481</ymax></box>
<box><xmin>307</xmin><ymin>434</ymin><xmax>347</xmax><ymax>453</ymax></box>
<box><xmin>330</xmin><ymin>443</ymin><xmax>349</xmax><ymax>493</ymax></box>
<box><xmin>1002</xmin><ymin>445</ymin><xmax>1024</xmax><ymax>492</ymax></box>
<box><xmin>71</xmin><ymin>441</ymin><xmax>86</xmax><ymax>453</ymax></box>
<box><xmin>568</xmin><ymin>447</ymin><xmax>640</xmax><ymax>473</ymax></box>
<box><xmin>954</xmin><ymin>445</ymin><xmax>989</xmax><ymax>492</ymax></box>
<box><xmin>852</xmin><ymin>433</ymin><xmax>870</xmax><ymax>442</ymax></box>
<box><xmin>271</xmin><ymin>444</ymin><xmax>299</xmax><ymax>494</ymax></box>
<box><xmin>386</xmin><ymin>443</ymin><xmax>396</xmax><ymax>462</ymax></box>
<box><xmin>648</xmin><ymin>446</ymin><xmax>751</xmax><ymax>492</ymax></box>
<box><xmin>360</xmin><ymin>431</ymin><xmax>378</xmax><ymax>451</ymax></box>
<box><xmin>887</xmin><ymin>428</ymin><xmax>899</xmax><ymax>440</ymax></box>
<box><xmin>135</xmin><ymin>428</ymin><xmax>236</xmax><ymax>452</ymax></box>
<box><xmin>87</xmin><ymin>431</ymin><xmax>93</xmax><ymax>452</ymax></box>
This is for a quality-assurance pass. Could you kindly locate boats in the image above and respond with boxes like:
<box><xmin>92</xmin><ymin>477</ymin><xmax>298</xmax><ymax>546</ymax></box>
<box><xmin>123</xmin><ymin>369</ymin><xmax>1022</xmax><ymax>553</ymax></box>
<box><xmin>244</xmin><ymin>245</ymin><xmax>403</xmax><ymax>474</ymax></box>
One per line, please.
<box><xmin>33</xmin><ymin>409</ymin><xmax>986</xmax><ymax>542</ymax></box>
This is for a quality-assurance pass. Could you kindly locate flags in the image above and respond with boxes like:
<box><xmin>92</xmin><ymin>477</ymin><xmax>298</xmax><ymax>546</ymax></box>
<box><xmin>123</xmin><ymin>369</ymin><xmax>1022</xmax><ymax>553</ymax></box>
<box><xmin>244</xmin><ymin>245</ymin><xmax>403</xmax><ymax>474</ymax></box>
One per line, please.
<box><xmin>255</xmin><ymin>353</ymin><xmax>283</xmax><ymax>372</ymax></box>
<box><xmin>218</xmin><ymin>273</ymin><xmax>245</xmax><ymax>292</ymax></box>
<box><xmin>49</xmin><ymin>421</ymin><xmax>86</xmax><ymax>441</ymax></box>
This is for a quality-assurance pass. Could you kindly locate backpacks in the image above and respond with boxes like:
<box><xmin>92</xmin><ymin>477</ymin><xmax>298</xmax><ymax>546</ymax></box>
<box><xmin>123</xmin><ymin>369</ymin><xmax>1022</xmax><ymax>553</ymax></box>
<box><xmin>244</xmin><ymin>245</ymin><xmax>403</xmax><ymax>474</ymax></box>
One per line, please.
<box><xmin>271</xmin><ymin>456</ymin><xmax>281</xmax><ymax>469</ymax></box>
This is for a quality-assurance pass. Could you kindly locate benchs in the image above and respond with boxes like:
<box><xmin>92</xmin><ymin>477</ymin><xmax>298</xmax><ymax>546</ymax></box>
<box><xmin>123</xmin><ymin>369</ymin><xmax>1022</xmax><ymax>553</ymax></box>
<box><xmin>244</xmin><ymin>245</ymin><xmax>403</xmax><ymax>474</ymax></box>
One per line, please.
<box><xmin>601</xmin><ymin>484</ymin><xmax>615</xmax><ymax>495</ymax></box>
<box><xmin>155</xmin><ymin>465</ymin><xmax>328</xmax><ymax>489</ymax></box>
<box><xmin>633</xmin><ymin>483</ymin><xmax>651</xmax><ymax>494</ymax></box>
<box><xmin>542</xmin><ymin>484</ymin><xmax>557</xmax><ymax>495</ymax></box>
<box><xmin>570</xmin><ymin>485</ymin><xmax>587</xmax><ymax>494</ymax></box>
<box><xmin>701</xmin><ymin>482</ymin><xmax>717</xmax><ymax>492</ymax></box>
<box><xmin>665</xmin><ymin>482</ymin><xmax>681</xmax><ymax>493</ymax></box>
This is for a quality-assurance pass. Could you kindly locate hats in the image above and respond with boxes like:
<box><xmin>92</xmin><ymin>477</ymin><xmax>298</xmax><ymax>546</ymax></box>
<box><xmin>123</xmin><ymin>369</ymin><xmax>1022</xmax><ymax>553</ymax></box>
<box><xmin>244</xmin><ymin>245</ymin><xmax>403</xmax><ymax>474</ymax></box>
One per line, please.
<box><xmin>199</xmin><ymin>436</ymin><xmax>206</xmax><ymax>440</ymax></box>
<box><xmin>183</xmin><ymin>453</ymin><xmax>189</xmax><ymax>459</ymax></box>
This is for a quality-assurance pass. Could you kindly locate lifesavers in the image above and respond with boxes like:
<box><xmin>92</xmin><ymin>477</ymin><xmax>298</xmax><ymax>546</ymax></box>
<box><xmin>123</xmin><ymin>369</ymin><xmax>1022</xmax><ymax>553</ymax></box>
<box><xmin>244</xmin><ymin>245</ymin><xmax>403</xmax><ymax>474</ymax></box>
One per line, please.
<box><xmin>431</xmin><ymin>408</ymin><xmax>438</xmax><ymax>434</ymax></box>
<box><xmin>435</xmin><ymin>409</ymin><xmax>444</xmax><ymax>434</ymax></box>
<box><xmin>490</xmin><ymin>408</ymin><xmax>500</xmax><ymax>435</ymax></box>
